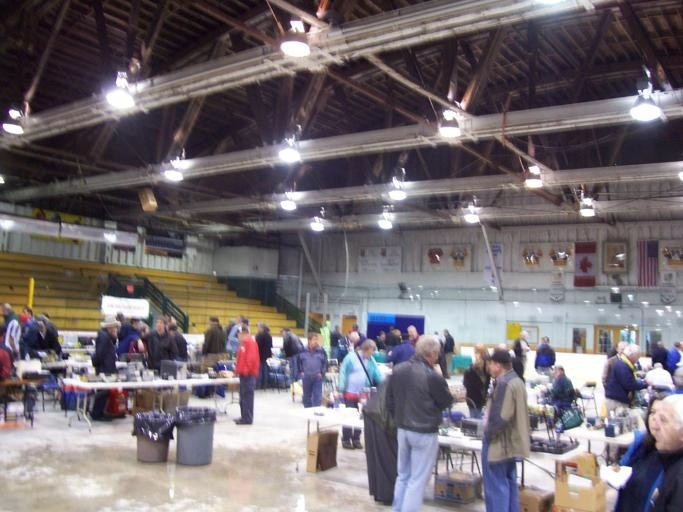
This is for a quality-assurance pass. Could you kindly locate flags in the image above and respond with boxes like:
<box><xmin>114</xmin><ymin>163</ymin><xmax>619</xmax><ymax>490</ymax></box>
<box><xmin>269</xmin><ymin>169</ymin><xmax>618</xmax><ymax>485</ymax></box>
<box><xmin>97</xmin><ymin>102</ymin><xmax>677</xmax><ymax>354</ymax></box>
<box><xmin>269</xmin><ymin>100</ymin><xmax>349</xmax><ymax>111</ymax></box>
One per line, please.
<box><xmin>573</xmin><ymin>240</ymin><xmax>596</xmax><ymax>286</ymax></box>
<box><xmin>635</xmin><ymin>239</ymin><xmax>657</xmax><ymax>287</ymax></box>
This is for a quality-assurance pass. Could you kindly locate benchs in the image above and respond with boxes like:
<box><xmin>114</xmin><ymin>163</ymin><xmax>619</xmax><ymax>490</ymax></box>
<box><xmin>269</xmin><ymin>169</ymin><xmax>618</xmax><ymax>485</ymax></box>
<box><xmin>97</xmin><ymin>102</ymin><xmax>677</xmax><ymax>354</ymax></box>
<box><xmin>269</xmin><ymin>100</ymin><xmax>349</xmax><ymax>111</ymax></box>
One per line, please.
<box><xmin>1</xmin><ymin>249</ymin><xmax>331</xmax><ymax>340</ymax></box>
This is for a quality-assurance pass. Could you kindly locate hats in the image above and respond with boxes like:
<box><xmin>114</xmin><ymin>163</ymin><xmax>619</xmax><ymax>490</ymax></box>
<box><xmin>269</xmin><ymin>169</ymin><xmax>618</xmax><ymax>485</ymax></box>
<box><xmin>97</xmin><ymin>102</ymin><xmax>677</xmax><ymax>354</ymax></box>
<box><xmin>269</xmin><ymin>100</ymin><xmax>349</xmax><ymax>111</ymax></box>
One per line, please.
<box><xmin>483</xmin><ymin>349</ymin><xmax>513</xmax><ymax>362</ymax></box>
<box><xmin>100</xmin><ymin>315</ymin><xmax>122</xmax><ymax>329</ymax></box>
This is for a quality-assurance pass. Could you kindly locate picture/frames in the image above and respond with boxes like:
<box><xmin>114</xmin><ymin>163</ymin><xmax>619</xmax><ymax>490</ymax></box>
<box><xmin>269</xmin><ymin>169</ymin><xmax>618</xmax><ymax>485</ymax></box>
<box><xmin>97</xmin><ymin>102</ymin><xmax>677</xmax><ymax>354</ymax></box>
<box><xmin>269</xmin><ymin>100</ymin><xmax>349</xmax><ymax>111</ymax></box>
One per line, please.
<box><xmin>600</xmin><ymin>236</ymin><xmax>631</xmax><ymax>274</ymax></box>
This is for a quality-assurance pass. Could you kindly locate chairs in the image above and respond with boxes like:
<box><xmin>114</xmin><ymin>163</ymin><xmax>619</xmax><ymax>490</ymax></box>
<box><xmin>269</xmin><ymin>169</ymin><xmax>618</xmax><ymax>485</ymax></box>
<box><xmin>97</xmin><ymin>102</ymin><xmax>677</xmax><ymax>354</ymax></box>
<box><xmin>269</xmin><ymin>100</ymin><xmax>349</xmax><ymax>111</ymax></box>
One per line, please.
<box><xmin>1</xmin><ymin>340</ymin><xmax>682</xmax><ymax>512</ymax></box>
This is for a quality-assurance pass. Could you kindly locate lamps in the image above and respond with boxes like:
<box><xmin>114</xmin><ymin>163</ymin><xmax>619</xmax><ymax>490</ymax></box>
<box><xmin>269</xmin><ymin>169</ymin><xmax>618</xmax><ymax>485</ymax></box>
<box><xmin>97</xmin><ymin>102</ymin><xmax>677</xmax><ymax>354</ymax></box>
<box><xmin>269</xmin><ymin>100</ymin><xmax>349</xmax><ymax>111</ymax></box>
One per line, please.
<box><xmin>279</xmin><ymin>9</ymin><xmax>312</xmax><ymax>60</ymax></box>
<box><xmin>105</xmin><ymin>66</ymin><xmax>137</xmax><ymax>111</ymax></box>
<box><xmin>626</xmin><ymin>88</ymin><xmax>661</xmax><ymax>122</ymax></box>
<box><xmin>1</xmin><ymin>101</ymin><xmax>28</xmax><ymax>137</ymax></box>
<box><xmin>279</xmin><ymin>105</ymin><xmax>603</xmax><ymax>227</ymax></box>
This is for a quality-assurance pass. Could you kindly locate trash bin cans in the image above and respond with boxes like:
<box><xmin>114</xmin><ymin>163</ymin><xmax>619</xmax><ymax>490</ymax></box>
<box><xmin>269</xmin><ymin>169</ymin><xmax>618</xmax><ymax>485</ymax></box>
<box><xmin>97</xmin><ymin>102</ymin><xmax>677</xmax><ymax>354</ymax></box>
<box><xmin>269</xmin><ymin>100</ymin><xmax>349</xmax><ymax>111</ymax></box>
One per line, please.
<box><xmin>132</xmin><ymin>412</ymin><xmax>175</xmax><ymax>462</ymax></box>
<box><xmin>175</xmin><ymin>406</ymin><xmax>216</xmax><ymax>465</ymax></box>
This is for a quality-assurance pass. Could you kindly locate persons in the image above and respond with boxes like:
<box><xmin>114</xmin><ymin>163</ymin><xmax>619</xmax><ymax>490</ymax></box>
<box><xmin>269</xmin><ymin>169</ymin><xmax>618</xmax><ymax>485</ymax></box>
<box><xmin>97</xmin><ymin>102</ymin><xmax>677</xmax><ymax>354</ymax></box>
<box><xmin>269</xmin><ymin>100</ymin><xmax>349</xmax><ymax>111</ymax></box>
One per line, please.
<box><xmin>613</xmin><ymin>391</ymin><xmax>683</xmax><ymax>512</ymax></box>
<box><xmin>390</xmin><ymin>334</ymin><xmax>453</xmax><ymax>512</ymax></box>
<box><xmin>601</xmin><ymin>340</ymin><xmax>683</xmax><ymax>410</ymax></box>
<box><xmin>0</xmin><ymin>302</ymin><xmax>61</xmax><ymax>421</ymax></box>
<box><xmin>442</xmin><ymin>329</ymin><xmax>456</xmax><ymax>353</ymax></box>
<box><xmin>479</xmin><ymin>349</ymin><xmax>531</xmax><ymax>512</ymax></box>
<box><xmin>466</xmin><ymin>330</ymin><xmax>575</xmax><ymax>418</ymax></box>
<box><xmin>200</xmin><ymin>315</ymin><xmax>419</xmax><ymax>450</ymax></box>
<box><xmin>93</xmin><ymin>312</ymin><xmax>187</xmax><ymax>421</ymax></box>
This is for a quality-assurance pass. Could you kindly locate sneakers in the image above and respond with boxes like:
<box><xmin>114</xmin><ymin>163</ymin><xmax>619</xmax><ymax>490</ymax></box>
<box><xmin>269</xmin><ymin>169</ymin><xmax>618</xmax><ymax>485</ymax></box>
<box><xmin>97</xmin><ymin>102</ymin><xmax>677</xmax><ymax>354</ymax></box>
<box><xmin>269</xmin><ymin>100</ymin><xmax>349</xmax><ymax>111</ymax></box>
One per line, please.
<box><xmin>233</xmin><ymin>417</ymin><xmax>253</xmax><ymax>425</ymax></box>
<box><xmin>341</xmin><ymin>438</ymin><xmax>363</xmax><ymax>450</ymax></box>
